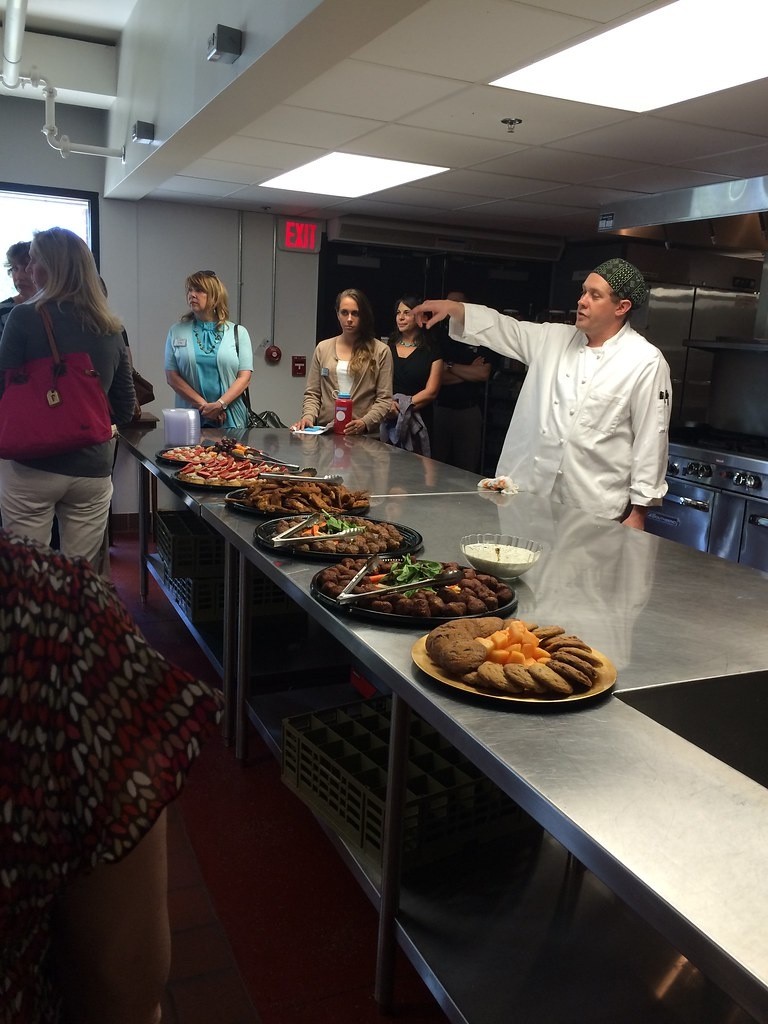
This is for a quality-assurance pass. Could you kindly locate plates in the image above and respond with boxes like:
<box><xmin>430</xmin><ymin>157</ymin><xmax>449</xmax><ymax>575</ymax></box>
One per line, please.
<box><xmin>311</xmin><ymin>559</ymin><xmax>520</xmax><ymax>628</ymax></box>
<box><xmin>254</xmin><ymin>514</ymin><xmax>423</xmax><ymax>564</ymax></box>
<box><xmin>411</xmin><ymin>634</ymin><xmax>617</xmax><ymax>703</ymax></box>
<box><xmin>171</xmin><ymin>469</ymin><xmax>248</xmax><ymax>492</ymax></box>
<box><xmin>155</xmin><ymin>444</ymin><xmax>210</xmax><ymax>466</ymax></box>
<box><xmin>224</xmin><ymin>488</ymin><xmax>370</xmax><ymax>520</ymax></box>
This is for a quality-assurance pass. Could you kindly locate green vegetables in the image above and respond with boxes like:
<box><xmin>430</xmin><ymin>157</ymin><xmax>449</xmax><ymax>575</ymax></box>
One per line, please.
<box><xmin>378</xmin><ymin>553</ymin><xmax>446</xmax><ymax>594</ymax></box>
<box><xmin>317</xmin><ymin>508</ymin><xmax>361</xmax><ymax>532</ymax></box>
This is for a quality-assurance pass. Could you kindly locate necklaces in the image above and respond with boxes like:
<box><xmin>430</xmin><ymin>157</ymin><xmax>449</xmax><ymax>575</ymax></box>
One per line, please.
<box><xmin>193</xmin><ymin>323</ymin><xmax>221</xmax><ymax>354</ymax></box>
<box><xmin>399</xmin><ymin>340</ymin><xmax>423</xmax><ymax>348</ymax></box>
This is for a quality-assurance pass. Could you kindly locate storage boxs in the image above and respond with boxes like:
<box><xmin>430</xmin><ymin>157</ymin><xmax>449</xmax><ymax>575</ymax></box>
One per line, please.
<box><xmin>160</xmin><ymin>565</ymin><xmax>300</xmax><ymax>620</ymax></box>
<box><xmin>150</xmin><ymin>509</ymin><xmax>225</xmax><ymax>575</ymax></box>
<box><xmin>283</xmin><ymin>692</ymin><xmax>544</xmax><ymax>874</ymax></box>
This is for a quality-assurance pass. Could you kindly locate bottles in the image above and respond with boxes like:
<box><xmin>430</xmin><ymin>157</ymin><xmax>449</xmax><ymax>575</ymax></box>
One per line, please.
<box><xmin>331</xmin><ymin>389</ymin><xmax>353</xmax><ymax>435</ymax></box>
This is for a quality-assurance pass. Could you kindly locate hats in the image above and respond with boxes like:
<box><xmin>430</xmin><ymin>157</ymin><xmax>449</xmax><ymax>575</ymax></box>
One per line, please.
<box><xmin>594</xmin><ymin>258</ymin><xmax>650</xmax><ymax>310</ymax></box>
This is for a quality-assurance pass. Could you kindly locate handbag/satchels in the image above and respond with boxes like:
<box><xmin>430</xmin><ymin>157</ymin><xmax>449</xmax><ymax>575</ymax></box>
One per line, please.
<box><xmin>0</xmin><ymin>303</ymin><xmax>112</xmax><ymax>458</ymax></box>
<box><xmin>246</xmin><ymin>411</ymin><xmax>287</xmax><ymax>429</ymax></box>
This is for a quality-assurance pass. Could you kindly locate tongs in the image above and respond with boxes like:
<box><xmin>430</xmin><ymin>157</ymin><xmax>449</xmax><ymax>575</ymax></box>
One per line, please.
<box><xmin>234</xmin><ymin>449</ymin><xmax>300</xmax><ymax>471</ymax></box>
<box><xmin>335</xmin><ymin>551</ymin><xmax>465</xmax><ymax>605</ymax></box>
<box><xmin>258</xmin><ymin>467</ymin><xmax>344</xmax><ymax>486</ymax></box>
<box><xmin>269</xmin><ymin>512</ymin><xmax>365</xmax><ymax>548</ymax></box>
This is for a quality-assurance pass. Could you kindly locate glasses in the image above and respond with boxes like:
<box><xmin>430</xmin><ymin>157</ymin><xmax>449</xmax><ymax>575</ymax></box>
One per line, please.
<box><xmin>200</xmin><ymin>271</ymin><xmax>216</xmax><ymax>279</ymax></box>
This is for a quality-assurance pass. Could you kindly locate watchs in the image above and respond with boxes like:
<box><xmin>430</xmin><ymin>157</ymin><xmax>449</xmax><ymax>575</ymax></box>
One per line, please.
<box><xmin>218</xmin><ymin>399</ymin><xmax>227</xmax><ymax>410</ymax></box>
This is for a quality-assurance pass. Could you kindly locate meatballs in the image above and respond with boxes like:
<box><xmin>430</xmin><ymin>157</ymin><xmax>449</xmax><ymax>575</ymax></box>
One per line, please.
<box><xmin>274</xmin><ymin>515</ymin><xmax>404</xmax><ymax>554</ymax></box>
<box><xmin>320</xmin><ymin>557</ymin><xmax>513</xmax><ymax>617</ymax></box>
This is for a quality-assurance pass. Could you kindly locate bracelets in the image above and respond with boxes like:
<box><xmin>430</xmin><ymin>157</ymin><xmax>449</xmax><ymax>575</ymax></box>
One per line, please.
<box><xmin>446</xmin><ymin>361</ymin><xmax>454</xmax><ymax>372</ymax></box>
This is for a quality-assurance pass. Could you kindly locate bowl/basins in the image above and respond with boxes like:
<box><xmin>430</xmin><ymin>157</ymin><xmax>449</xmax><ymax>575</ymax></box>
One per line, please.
<box><xmin>459</xmin><ymin>534</ymin><xmax>543</xmax><ymax>581</ymax></box>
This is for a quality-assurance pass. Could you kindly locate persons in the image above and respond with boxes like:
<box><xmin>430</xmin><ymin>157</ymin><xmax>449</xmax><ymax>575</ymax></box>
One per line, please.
<box><xmin>0</xmin><ymin>228</ymin><xmax>136</xmax><ymax>587</ymax></box>
<box><xmin>0</xmin><ymin>242</ymin><xmax>38</xmax><ymax>338</ymax></box>
<box><xmin>428</xmin><ymin>289</ymin><xmax>492</xmax><ymax>472</ymax></box>
<box><xmin>290</xmin><ymin>288</ymin><xmax>392</xmax><ymax>435</ymax></box>
<box><xmin>0</xmin><ymin>528</ymin><xmax>226</xmax><ymax>1024</ymax></box>
<box><xmin>379</xmin><ymin>293</ymin><xmax>444</xmax><ymax>459</ymax></box>
<box><xmin>408</xmin><ymin>258</ymin><xmax>673</xmax><ymax>531</ymax></box>
<box><xmin>165</xmin><ymin>270</ymin><xmax>254</xmax><ymax>428</ymax></box>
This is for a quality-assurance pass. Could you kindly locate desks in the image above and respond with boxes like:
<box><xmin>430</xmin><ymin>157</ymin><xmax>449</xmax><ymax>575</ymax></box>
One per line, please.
<box><xmin>112</xmin><ymin>429</ymin><xmax>768</xmax><ymax>1024</ymax></box>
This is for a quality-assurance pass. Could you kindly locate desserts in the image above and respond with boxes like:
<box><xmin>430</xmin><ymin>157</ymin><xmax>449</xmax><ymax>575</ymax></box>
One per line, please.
<box><xmin>174</xmin><ymin>456</ymin><xmax>287</xmax><ymax>485</ymax></box>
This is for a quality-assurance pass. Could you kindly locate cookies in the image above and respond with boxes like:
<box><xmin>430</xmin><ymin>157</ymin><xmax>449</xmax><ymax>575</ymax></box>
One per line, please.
<box><xmin>425</xmin><ymin>615</ymin><xmax>603</xmax><ymax>696</ymax></box>
